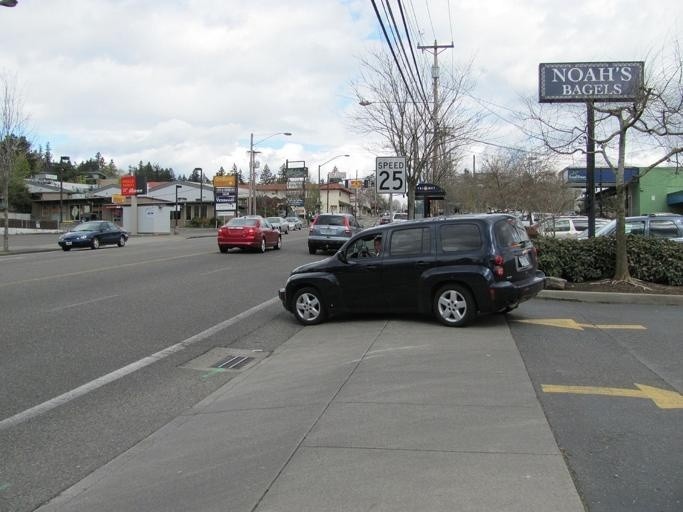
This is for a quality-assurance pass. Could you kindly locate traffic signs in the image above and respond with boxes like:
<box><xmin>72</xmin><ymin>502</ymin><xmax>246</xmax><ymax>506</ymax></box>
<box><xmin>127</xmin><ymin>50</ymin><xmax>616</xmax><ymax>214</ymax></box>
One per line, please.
<box><xmin>375</xmin><ymin>156</ymin><xmax>406</xmax><ymax>195</ymax></box>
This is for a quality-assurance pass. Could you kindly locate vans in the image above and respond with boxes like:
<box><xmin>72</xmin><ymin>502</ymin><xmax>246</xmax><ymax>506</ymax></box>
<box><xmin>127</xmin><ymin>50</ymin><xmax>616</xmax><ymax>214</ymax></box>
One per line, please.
<box><xmin>273</xmin><ymin>206</ymin><xmax>550</xmax><ymax>334</ymax></box>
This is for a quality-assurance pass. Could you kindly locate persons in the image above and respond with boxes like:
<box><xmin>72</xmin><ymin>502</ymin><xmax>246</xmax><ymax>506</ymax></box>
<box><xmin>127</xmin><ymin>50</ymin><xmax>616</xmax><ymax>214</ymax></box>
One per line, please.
<box><xmin>371</xmin><ymin>236</ymin><xmax>383</xmax><ymax>257</ymax></box>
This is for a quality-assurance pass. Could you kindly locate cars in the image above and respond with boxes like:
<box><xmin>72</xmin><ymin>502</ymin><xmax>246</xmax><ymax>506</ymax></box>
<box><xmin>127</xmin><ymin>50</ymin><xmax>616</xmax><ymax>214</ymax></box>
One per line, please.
<box><xmin>308</xmin><ymin>213</ymin><xmax>365</xmax><ymax>254</ymax></box>
<box><xmin>215</xmin><ymin>214</ymin><xmax>283</xmax><ymax>255</ymax></box>
<box><xmin>379</xmin><ymin>212</ymin><xmax>409</xmax><ymax>224</ymax></box>
<box><xmin>55</xmin><ymin>218</ymin><xmax>130</xmax><ymax>255</ymax></box>
<box><xmin>517</xmin><ymin>211</ymin><xmax>611</xmax><ymax>242</ymax></box>
<box><xmin>267</xmin><ymin>218</ymin><xmax>291</xmax><ymax>234</ymax></box>
<box><xmin>79</xmin><ymin>212</ymin><xmax>96</xmax><ymax>223</ymax></box>
<box><xmin>286</xmin><ymin>217</ymin><xmax>303</xmax><ymax>231</ymax></box>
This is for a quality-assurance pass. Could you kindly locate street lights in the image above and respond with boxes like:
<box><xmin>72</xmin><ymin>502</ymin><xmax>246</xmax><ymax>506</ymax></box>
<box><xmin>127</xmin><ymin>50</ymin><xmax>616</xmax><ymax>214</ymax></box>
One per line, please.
<box><xmin>174</xmin><ymin>184</ymin><xmax>182</xmax><ymax>229</ymax></box>
<box><xmin>58</xmin><ymin>155</ymin><xmax>70</xmax><ymax>223</ymax></box>
<box><xmin>357</xmin><ymin>98</ymin><xmax>438</xmax><ymax>216</ymax></box>
<box><xmin>317</xmin><ymin>154</ymin><xmax>350</xmax><ymax>216</ymax></box>
<box><xmin>0</xmin><ymin>0</ymin><xmax>19</xmax><ymax>9</ymax></box>
<box><xmin>246</xmin><ymin>131</ymin><xmax>294</xmax><ymax>216</ymax></box>
<box><xmin>194</xmin><ymin>166</ymin><xmax>203</xmax><ymax>219</ymax></box>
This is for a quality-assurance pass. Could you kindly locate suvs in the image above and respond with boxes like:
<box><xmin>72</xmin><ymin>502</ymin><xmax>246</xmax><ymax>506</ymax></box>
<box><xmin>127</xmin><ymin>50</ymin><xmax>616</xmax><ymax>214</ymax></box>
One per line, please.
<box><xmin>578</xmin><ymin>213</ymin><xmax>683</xmax><ymax>247</ymax></box>
